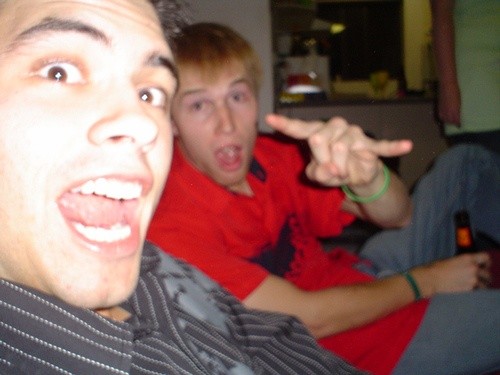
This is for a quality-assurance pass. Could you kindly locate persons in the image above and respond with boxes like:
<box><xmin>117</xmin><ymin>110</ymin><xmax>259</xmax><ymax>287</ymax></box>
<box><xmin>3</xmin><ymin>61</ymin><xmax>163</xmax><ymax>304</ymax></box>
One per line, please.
<box><xmin>146</xmin><ymin>25</ymin><xmax>500</xmax><ymax>375</ymax></box>
<box><xmin>1</xmin><ymin>0</ymin><xmax>372</xmax><ymax>375</ymax></box>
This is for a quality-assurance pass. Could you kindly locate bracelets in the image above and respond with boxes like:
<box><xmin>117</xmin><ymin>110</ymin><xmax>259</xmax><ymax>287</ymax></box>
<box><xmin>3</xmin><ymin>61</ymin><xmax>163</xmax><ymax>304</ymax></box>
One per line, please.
<box><xmin>341</xmin><ymin>159</ymin><xmax>391</xmax><ymax>203</ymax></box>
<box><xmin>401</xmin><ymin>269</ymin><xmax>421</xmax><ymax>302</ymax></box>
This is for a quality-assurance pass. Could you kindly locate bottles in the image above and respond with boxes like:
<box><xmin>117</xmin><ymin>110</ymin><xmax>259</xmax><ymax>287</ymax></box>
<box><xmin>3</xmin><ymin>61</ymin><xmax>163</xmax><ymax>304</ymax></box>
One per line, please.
<box><xmin>454</xmin><ymin>210</ymin><xmax>476</xmax><ymax>257</ymax></box>
